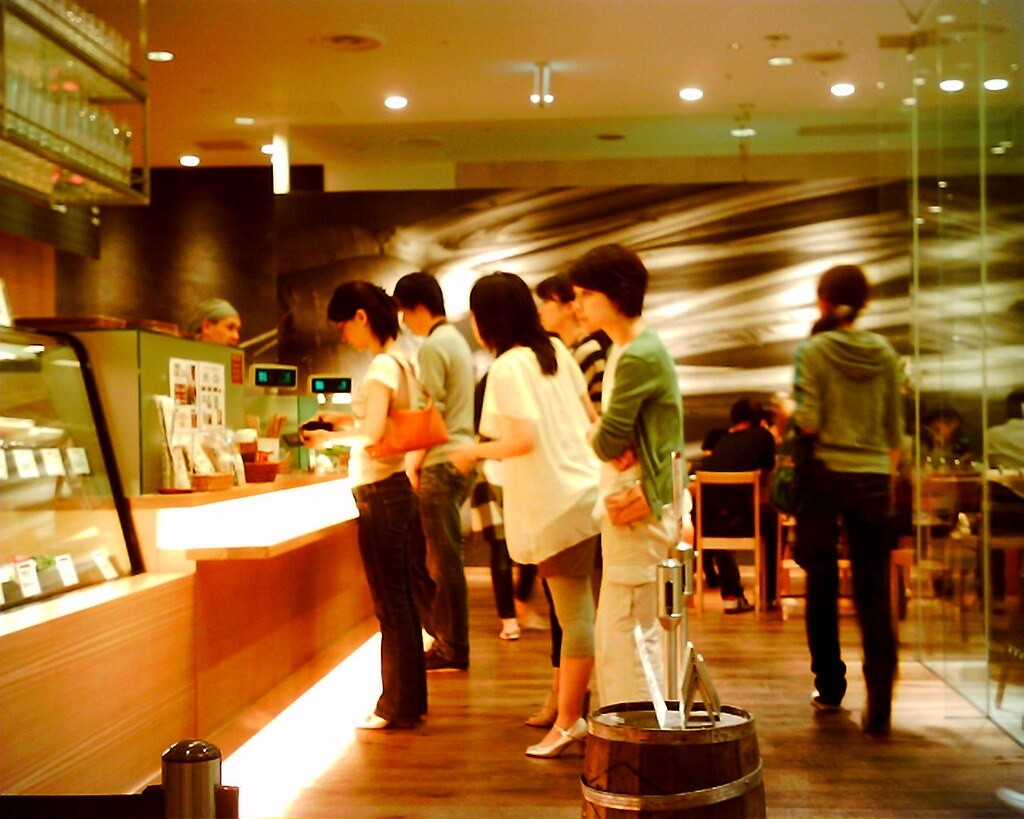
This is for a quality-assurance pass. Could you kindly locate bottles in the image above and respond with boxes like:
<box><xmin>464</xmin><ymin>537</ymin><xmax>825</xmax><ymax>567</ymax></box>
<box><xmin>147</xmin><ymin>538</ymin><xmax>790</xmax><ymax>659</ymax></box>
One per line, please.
<box><xmin>3</xmin><ymin>0</ymin><xmax>131</xmax><ymax>200</ymax></box>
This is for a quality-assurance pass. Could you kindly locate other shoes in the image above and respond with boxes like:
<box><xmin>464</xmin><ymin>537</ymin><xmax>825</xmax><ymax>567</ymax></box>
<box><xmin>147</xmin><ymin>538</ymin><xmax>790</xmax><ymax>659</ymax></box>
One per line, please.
<box><xmin>425</xmin><ymin>650</ymin><xmax>469</xmax><ymax>672</ymax></box>
<box><xmin>514</xmin><ymin>600</ymin><xmax>550</xmax><ymax>630</ymax></box>
<box><xmin>811</xmin><ymin>692</ymin><xmax>838</xmax><ymax>712</ymax></box>
<box><xmin>502</xmin><ymin>619</ymin><xmax>524</xmax><ymax>639</ymax></box>
<box><xmin>723</xmin><ymin>595</ymin><xmax>756</xmax><ymax>614</ymax></box>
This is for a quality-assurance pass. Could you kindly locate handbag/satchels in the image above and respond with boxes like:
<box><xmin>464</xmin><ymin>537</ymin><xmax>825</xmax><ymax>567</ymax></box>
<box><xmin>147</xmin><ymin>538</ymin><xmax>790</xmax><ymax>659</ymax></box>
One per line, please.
<box><xmin>607</xmin><ymin>485</ymin><xmax>653</xmax><ymax>527</ymax></box>
<box><xmin>366</xmin><ymin>355</ymin><xmax>450</xmax><ymax>458</ymax></box>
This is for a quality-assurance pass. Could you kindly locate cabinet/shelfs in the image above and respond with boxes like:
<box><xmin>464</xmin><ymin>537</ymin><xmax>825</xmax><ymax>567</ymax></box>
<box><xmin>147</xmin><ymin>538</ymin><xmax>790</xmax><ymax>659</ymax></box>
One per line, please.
<box><xmin>0</xmin><ymin>0</ymin><xmax>150</xmax><ymax>205</ymax></box>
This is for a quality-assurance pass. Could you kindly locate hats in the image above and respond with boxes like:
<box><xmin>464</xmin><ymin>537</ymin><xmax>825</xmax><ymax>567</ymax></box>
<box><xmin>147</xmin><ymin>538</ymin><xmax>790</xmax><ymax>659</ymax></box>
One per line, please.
<box><xmin>191</xmin><ymin>299</ymin><xmax>236</xmax><ymax>333</ymax></box>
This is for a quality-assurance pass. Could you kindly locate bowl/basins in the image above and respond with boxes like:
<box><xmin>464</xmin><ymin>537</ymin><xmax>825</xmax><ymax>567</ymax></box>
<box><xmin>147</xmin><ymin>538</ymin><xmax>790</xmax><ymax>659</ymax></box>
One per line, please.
<box><xmin>244</xmin><ymin>462</ymin><xmax>280</xmax><ymax>483</ymax></box>
<box><xmin>193</xmin><ymin>472</ymin><xmax>236</xmax><ymax>490</ymax></box>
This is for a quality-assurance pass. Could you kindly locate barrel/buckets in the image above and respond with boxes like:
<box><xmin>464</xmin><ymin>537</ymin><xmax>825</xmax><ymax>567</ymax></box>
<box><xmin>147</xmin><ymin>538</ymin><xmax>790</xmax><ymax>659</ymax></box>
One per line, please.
<box><xmin>581</xmin><ymin>702</ymin><xmax>766</xmax><ymax>819</ymax></box>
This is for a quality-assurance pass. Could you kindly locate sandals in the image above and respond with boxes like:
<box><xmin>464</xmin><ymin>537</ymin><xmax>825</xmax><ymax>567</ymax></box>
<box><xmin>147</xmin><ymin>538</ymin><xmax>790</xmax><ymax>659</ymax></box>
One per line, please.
<box><xmin>528</xmin><ymin>706</ymin><xmax>559</xmax><ymax>728</ymax></box>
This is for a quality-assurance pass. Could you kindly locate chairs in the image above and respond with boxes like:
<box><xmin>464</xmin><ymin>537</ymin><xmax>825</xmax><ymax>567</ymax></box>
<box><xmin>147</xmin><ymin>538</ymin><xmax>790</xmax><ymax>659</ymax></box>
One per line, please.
<box><xmin>962</xmin><ymin>530</ymin><xmax>1024</xmax><ymax>731</ymax></box>
<box><xmin>694</xmin><ymin>470</ymin><xmax>768</xmax><ymax>617</ymax></box>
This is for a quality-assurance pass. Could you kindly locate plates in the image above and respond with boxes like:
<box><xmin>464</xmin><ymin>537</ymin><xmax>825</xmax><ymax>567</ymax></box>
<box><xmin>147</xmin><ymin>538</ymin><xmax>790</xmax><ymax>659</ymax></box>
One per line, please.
<box><xmin>157</xmin><ymin>488</ymin><xmax>194</xmax><ymax>494</ymax></box>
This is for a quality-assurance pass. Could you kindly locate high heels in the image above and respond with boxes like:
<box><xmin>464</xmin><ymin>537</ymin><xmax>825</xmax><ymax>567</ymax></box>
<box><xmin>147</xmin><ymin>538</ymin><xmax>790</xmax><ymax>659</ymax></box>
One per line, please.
<box><xmin>356</xmin><ymin>712</ymin><xmax>390</xmax><ymax>730</ymax></box>
<box><xmin>526</xmin><ymin>718</ymin><xmax>588</xmax><ymax>756</ymax></box>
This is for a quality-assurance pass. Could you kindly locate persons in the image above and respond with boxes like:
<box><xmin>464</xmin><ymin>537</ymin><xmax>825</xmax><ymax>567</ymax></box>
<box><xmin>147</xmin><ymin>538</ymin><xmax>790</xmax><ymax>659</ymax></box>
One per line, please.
<box><xmin>391</xmin><ymin>271</ymin><xmax>478</xmax><ymax>674</ymax></box>
<box><xmin>186</xmin><ymin>297</ymin><xmax>242</xmax><ymax>349</ymax></box>
<box><xmin>445</xmin><ymin>241</ymin><xmax>687</xmax><ymax>757</ymax></box>
<box><xmin>774</xmin><ymin>265</ymin><xmax>906</xmax><ymax>737</ymax></box>
<box><xmin>694</xmin><ymin>392</ymin><xmax>783</xmax><ymax>614</ymax></box>
<box><xmin>899</xmin><ymin>384</ymin><xmax>1024</xmax><ymax>614</ymax></box>
<box><xmin>296</xmin><ymin>279</ymin><xmax>428</xmax><ymax>733</ymax></box>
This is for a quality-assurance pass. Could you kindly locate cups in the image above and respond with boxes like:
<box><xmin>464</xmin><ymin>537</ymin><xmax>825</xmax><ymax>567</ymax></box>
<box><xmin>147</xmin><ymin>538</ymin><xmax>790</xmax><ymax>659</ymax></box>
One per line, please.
<box><xmin>258</xmin><ymin>437</ymin><xmax>280</xmax><ymax>462</ymax></box>
<box><xmin>237</xmin><ymin>429</ymin><xmax>259</xmax><ymax>462</ymax></box>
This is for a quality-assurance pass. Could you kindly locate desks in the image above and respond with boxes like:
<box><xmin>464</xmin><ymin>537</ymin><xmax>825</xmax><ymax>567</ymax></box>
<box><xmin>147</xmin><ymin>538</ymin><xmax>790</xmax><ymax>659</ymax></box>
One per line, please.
<box><xmin>910</xmin><ymin>471</ymin><xmax>984</xmax><ymax>526</ymax></box>
